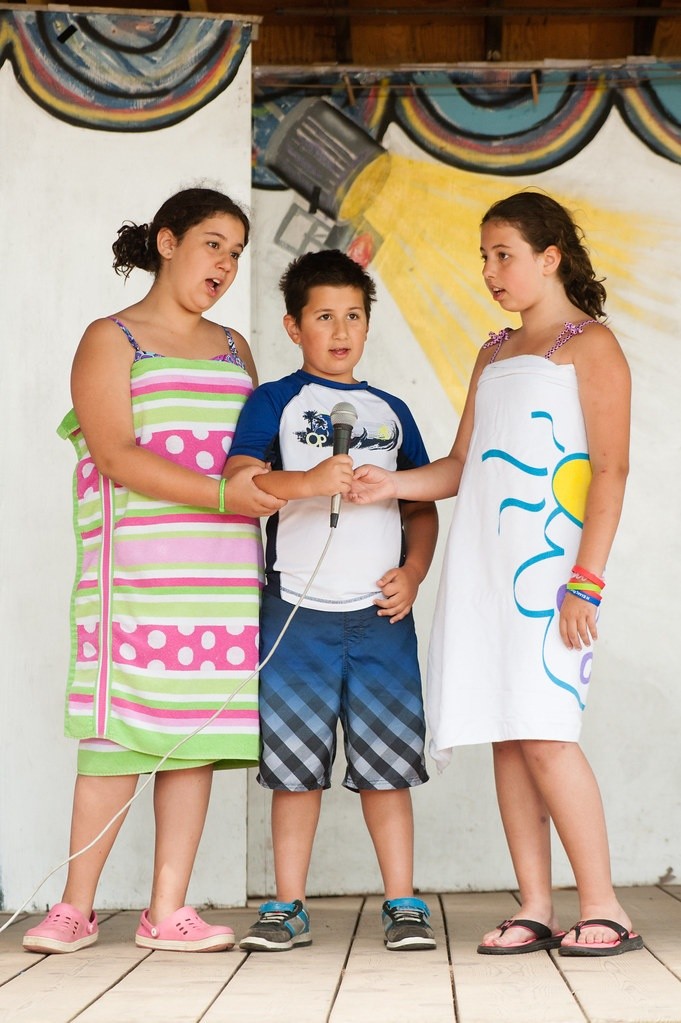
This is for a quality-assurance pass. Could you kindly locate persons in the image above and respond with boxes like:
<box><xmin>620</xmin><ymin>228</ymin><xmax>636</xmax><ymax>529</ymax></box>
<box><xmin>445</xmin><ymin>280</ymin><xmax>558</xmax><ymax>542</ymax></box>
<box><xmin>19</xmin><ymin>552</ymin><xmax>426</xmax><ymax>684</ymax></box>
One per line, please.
<box><xmin>345</xmin><ymin>191</ymin><xmax>644</xmax><ymax>957</ymax></box>
<box><xmin>21</xmin><ymin>187</ymin><xmax>288</xmax><ymax>953</ymax></box>
<box><xmin>220</xmin><ymin>252</ymin><xmax>441</xmax><ymax>953</ymax></box>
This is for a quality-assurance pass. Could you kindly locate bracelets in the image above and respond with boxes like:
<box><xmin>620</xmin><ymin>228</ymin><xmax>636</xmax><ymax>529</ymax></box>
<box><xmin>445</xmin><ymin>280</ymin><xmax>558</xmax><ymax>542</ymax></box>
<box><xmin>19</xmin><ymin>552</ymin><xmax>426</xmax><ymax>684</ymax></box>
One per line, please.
<box><xmin>219</xmin><ymin>479</ymin><xmax>227</xmax><ymax>513</ymax></box>
<box><xmin>566</xmin><ymin>566</ymin><xmax>605</xmax><ymax>606</ymax></box>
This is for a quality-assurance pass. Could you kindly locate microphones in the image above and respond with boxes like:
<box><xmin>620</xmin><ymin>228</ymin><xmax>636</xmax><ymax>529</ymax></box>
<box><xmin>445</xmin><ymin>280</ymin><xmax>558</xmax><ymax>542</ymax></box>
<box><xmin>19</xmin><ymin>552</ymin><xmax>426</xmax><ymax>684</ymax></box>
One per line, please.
<box><xmin>329</xmin><ymin>401</ymin><xmax>358</xmax><ymax>529</ymax></box>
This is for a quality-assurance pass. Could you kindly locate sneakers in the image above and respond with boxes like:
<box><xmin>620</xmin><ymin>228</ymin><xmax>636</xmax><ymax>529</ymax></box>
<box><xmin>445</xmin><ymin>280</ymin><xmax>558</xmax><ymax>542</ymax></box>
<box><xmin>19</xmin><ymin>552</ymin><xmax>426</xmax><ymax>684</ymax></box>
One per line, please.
<box><xmin>380</xmin><ymin>898</ymin><xmax>437</xmax><ymax>949</ymax></box>
<box><xmin>238</xmin><ymin>900</ymin><xmax>312</xmax><ymax>951</ymax></box>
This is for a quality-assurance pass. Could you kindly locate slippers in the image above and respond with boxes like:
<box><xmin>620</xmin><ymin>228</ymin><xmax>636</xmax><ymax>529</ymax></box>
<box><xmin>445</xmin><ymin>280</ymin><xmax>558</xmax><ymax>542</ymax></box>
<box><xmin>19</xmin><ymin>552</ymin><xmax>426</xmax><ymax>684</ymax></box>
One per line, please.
<box><xmin>558</xmin><ymin>919</ymin><xmax>643</xmax><ymax>955</ymax></box>
<box><xmin>477</xmin><ymin>919</ymin><xmax>568</xmax><ymax>954</ymax></box>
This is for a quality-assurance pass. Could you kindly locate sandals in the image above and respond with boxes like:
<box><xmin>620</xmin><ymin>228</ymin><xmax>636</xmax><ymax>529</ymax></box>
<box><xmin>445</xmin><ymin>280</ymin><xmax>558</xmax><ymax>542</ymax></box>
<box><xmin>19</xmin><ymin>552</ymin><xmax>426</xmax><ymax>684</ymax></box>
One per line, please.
<box><xmin>135</xmin><ymin>905</ymin><xmax>235</xmax><ymax>952</ymax></box>
<box><xmin>22</xmin><ymin>903</ymin><xmax>98</xmax><ymax>953</ymax></box>
